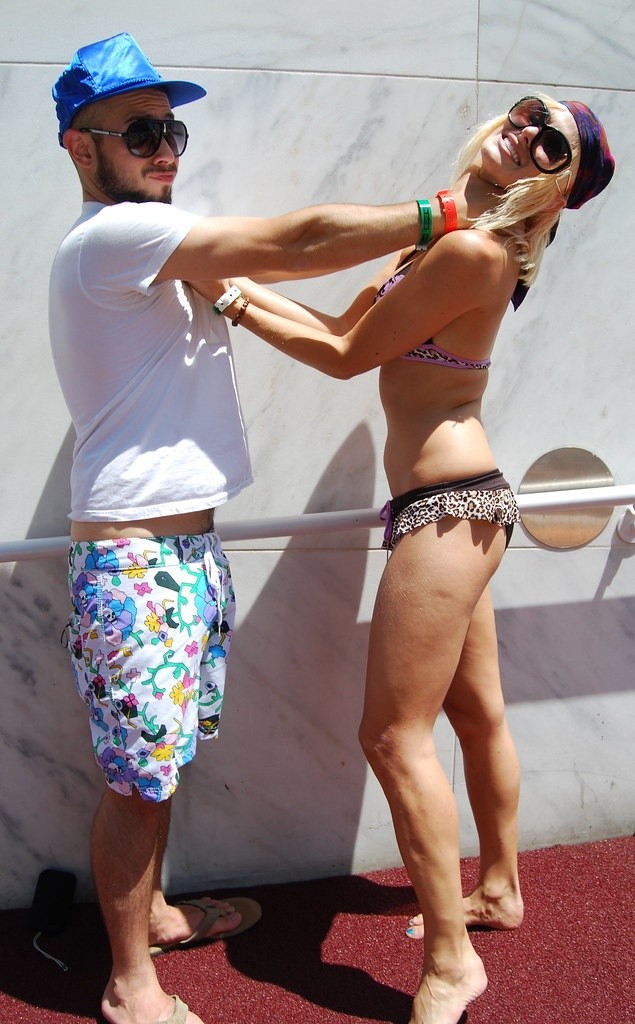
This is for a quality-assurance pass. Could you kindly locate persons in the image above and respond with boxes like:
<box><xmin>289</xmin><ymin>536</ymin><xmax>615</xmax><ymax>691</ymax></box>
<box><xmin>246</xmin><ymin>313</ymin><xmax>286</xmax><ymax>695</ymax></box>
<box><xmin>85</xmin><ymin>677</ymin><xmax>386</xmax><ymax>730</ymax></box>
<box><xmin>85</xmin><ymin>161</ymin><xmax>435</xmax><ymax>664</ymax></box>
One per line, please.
<box><xmin>185</xmin><ymin>94</ymin><xmax>613</xmax><ymax>1024</ymax></box>
<box><xmin>48</xmin><ymin>34</ymin><xmax>525</xmax><ymax>1024</ymax></box>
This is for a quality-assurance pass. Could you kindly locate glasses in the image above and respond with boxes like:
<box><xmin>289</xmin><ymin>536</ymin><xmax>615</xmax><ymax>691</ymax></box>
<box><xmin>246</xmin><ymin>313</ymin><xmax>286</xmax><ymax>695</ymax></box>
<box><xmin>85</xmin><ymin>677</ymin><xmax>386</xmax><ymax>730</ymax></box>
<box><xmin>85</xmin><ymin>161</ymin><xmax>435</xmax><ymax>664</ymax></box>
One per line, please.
<box><xmin>507</xmin><ymin>96</ymin><xmax>573</xmax><ymax>196</ymax></box>
<box><xmin>80</xmin><ymin>119</ymin><xmax>189</xmax><ymax>158</ymax></box>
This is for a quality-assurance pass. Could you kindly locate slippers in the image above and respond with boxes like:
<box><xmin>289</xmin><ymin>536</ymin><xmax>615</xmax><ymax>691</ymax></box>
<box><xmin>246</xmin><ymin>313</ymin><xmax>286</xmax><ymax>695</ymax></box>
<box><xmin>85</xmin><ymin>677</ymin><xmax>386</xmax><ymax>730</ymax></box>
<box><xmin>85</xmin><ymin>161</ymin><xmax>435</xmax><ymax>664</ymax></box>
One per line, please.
<box><xmin>166</xmin><ymin>995</ymin><xmax>205</xmax><ymax>1024</ymax></box>
<box><xmin>149</xmin><ymin>897</ymin><xmax>262</xmax><ymax>956</ymax></box>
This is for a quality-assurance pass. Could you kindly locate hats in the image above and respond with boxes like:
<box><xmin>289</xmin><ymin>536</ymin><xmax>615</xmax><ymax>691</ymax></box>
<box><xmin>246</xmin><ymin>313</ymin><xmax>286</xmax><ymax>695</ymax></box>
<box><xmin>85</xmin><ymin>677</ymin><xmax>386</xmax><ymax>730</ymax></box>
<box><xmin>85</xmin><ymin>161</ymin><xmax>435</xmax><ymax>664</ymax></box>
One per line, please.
<box><xmin>52</xmin><ymin>32</ymin><xmax>208</xmax><ymax>150</ymax></box>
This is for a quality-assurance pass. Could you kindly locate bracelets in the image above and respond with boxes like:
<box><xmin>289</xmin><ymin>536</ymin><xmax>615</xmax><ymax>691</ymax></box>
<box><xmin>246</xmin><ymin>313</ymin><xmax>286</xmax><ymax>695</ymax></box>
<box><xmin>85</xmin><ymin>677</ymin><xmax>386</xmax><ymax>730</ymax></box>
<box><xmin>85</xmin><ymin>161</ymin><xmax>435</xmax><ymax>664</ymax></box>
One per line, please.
<box><xmin>232</xmin><ymin>298</ymin><xmax>250</xmax><ymax>327</ymax></box>
<box><xmin>213</xmin><ymin>286</ymin><xmax>241</xmax><ymax>316</ymax></box>
<box><xmin>414</xmin><ymin>200</ymin><xmax>433</xmax><ymax>251</ymax></box>
<box><xmin>436</xmin><ymin>190</ymin><xmax>457</xmax><ymax>232</ymax></box>
